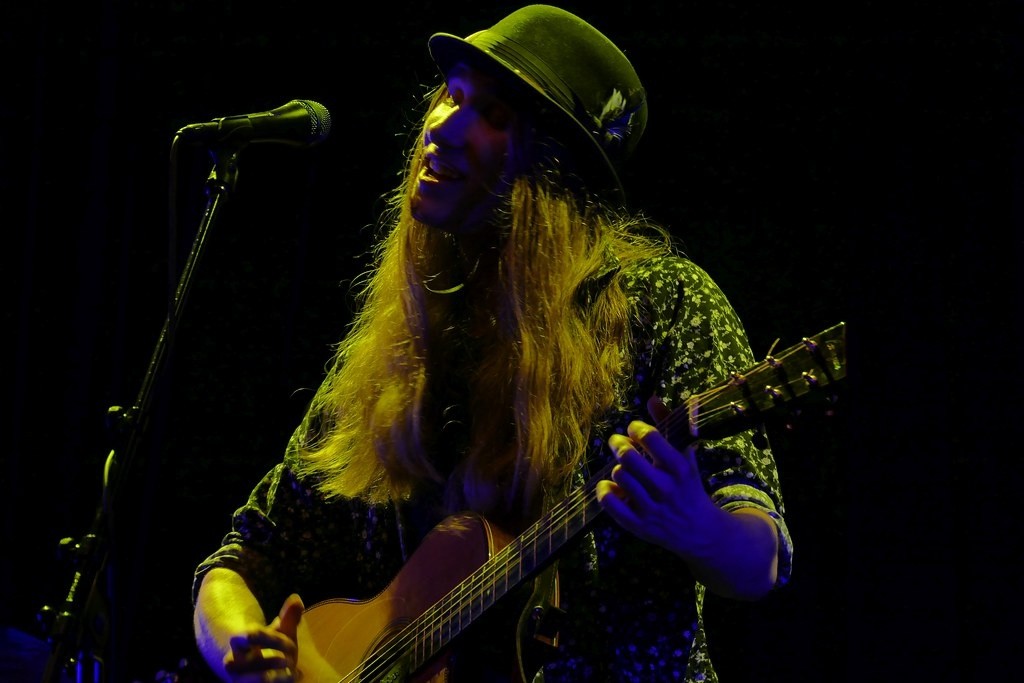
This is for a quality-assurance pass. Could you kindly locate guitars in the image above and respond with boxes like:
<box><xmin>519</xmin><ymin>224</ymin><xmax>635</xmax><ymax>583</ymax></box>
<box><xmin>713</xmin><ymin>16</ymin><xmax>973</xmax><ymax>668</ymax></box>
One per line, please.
<box><xmin>296</xmin><ymin>317</ymin><xmax>850</xmax><ymax>682</ymax></box>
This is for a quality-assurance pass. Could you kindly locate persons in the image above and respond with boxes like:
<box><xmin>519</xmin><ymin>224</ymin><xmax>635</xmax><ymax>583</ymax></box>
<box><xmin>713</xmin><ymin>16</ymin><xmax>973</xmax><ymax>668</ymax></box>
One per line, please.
<box><xmin>179</xmin><ymin>5</ymin><xmax>800</xmax><ymax>683</ymax></box>
<box><xmin>2</xmin><ymin>365</ymin><xmax>146</xmax><ymax>678</ymax></box>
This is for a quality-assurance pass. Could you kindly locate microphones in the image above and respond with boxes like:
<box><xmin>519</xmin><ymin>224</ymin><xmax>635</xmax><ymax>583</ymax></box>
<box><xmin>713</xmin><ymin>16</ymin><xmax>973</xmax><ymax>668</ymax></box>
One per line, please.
<box><xmin>176</xmin><ymin>99</ymin><xmax>331</xmax><ymax>148</ymax></box>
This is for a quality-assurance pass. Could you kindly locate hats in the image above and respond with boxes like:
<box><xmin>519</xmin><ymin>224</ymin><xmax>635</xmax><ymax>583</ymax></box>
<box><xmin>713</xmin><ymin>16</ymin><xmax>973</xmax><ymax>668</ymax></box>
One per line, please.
<box><xmin>430</xmin><ymin>3</ymin><xmax>648</xmax><ymax>210</ymax></box>
